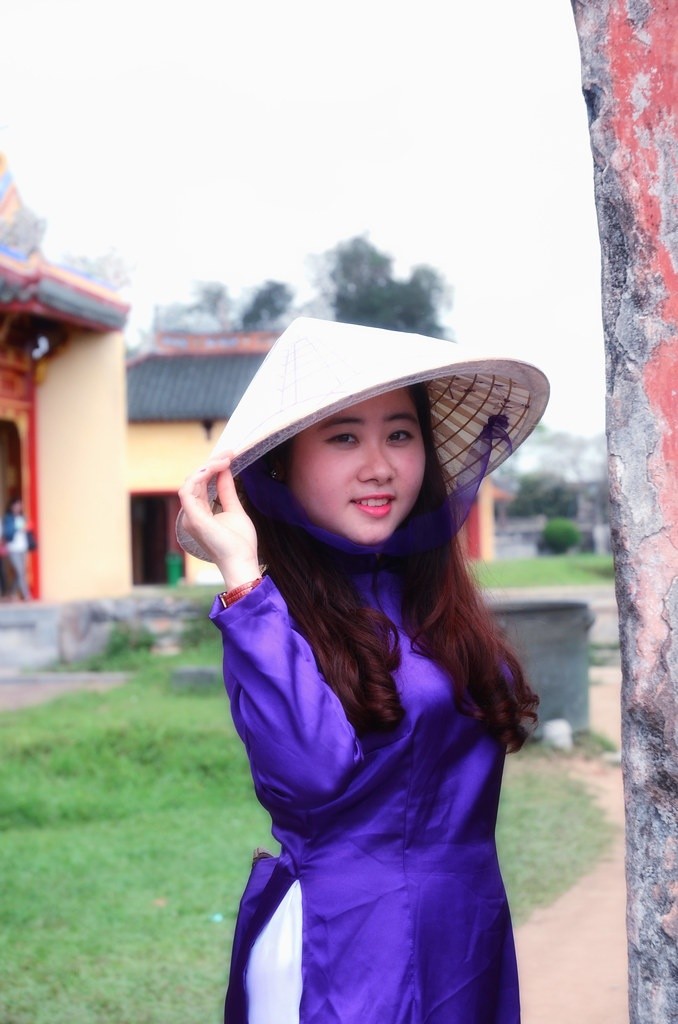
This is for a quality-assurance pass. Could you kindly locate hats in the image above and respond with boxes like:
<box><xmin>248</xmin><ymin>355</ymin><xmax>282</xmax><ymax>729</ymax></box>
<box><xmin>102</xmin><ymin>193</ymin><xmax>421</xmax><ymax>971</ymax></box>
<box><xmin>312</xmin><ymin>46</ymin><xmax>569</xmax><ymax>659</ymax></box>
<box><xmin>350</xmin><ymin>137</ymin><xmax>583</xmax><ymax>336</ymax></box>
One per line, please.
<box><xmin>174</xmin><ymin>317</ymin><xmax>551</xmax><ymax>564</ymax></box>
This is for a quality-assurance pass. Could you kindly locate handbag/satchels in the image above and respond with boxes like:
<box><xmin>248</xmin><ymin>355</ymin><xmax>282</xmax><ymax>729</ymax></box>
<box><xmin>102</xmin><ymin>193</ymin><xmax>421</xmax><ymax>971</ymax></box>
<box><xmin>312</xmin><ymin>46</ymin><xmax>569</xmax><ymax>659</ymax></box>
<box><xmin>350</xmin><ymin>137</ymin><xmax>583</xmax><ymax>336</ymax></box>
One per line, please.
<box><xmin>27</xmin><ymin>532</ymin><xmax>35</xmax><ymax>550</ymax></box>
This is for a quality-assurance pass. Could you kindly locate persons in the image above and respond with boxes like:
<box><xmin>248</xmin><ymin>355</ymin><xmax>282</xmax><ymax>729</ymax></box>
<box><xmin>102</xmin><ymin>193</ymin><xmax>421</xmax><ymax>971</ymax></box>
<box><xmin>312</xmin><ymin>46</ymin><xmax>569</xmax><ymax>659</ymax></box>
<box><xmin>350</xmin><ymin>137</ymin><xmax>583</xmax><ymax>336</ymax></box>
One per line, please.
<box><xmin>175</xmin><ymin>316</ymin><xmax>551</xmax><ymax>1024</ymax></box>
<box><xmin>0</xmin><ymin>498</ymin><xmax>36</xmax><ymax>602</ymax></box>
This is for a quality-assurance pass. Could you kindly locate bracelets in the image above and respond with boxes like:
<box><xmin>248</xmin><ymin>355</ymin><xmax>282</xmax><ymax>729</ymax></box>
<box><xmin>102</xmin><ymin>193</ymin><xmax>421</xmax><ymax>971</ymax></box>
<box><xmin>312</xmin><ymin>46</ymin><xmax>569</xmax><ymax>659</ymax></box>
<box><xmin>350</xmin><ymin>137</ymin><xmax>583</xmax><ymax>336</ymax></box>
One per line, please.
<box><xmin>217</xmin><ymin>577</ymin><xmax>264</xmax><ymax>608</ymax></box>
